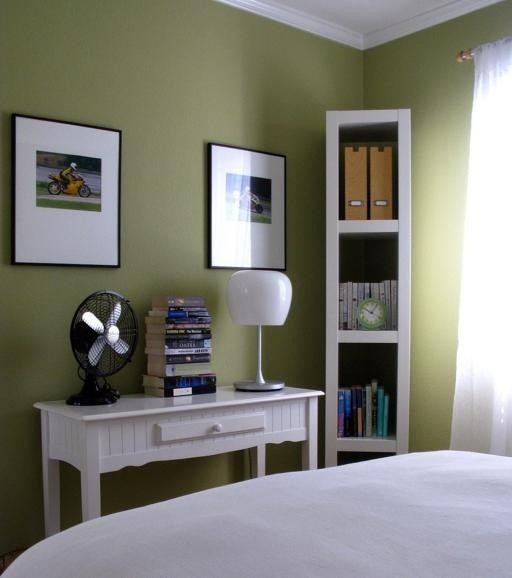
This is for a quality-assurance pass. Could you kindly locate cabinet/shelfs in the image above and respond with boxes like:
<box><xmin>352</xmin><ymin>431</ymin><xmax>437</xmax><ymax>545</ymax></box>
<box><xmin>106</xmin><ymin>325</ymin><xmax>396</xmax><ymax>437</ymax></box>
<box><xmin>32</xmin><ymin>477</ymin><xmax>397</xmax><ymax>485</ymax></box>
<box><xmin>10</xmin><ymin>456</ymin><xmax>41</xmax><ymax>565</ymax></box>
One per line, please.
<box><xmin>325</xmin><ymin>109</ymin><xmax>412</xmax><ymax>469</ymax></box>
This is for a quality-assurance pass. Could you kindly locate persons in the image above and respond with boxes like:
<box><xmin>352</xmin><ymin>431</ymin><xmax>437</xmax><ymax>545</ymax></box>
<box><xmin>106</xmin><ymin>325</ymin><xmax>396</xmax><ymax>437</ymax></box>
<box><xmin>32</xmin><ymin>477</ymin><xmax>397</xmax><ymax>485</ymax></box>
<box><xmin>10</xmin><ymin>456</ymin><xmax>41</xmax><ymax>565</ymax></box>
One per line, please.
<box><xmin>59</xmin><ymin>160</ymin><xmax>80</xmax><ymax>189</ymax></box>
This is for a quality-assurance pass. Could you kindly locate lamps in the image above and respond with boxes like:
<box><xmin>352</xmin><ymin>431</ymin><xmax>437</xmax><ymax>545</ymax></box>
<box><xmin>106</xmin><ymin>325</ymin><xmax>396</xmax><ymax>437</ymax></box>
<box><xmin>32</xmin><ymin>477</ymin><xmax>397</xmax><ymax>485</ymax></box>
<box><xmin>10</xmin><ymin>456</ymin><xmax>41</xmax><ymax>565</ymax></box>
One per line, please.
<box><xmin>226</xmin><ymin>270</ymin><xmax>293</xmax><ymax>392</ymax></box>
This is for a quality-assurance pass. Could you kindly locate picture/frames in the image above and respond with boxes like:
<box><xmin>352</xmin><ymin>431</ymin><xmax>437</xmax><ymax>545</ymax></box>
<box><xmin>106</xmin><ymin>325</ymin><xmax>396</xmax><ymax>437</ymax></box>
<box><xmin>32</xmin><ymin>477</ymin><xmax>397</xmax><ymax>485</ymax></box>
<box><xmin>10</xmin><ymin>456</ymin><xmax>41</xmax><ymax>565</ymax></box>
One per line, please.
<box><xmin>207</xmin><ymin>142</ymin><xmax>287</xmax><ymax>272</ymax></box>
<box><xmin>11</xmin><ymin>113</ymin><xmax>122</xmax><ymax>268</ymax></box>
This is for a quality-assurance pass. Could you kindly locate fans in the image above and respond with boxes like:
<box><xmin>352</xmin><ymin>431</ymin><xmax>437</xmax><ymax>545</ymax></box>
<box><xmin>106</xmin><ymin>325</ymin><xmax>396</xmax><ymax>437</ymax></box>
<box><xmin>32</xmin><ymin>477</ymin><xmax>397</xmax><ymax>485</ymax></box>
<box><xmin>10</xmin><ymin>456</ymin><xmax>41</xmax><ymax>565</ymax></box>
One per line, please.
<box><xmin>65</xmin><ymin>290</ymin><xmax>139</xmax><ymax>406</ymax></box>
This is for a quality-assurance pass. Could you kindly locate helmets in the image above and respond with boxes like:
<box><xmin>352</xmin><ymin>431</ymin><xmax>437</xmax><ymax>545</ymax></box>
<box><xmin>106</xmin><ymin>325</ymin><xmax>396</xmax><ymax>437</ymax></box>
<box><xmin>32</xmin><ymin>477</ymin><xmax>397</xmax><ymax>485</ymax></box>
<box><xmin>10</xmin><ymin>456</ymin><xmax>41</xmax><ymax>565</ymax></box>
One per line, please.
<box><xmin>70</xmin><ymin>162</ymin><xmax>79</xmax><ymax>171</ymax></box>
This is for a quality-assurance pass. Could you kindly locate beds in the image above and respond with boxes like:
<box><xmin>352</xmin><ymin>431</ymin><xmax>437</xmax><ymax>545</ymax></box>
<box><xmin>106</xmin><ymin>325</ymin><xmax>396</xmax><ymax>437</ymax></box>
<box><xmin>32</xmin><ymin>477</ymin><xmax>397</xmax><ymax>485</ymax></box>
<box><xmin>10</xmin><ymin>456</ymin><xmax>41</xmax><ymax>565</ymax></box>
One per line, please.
<box><xmin>0</xmin><ymin>450</ymin><xmax>512</xmax><ymax>578</ymax></box>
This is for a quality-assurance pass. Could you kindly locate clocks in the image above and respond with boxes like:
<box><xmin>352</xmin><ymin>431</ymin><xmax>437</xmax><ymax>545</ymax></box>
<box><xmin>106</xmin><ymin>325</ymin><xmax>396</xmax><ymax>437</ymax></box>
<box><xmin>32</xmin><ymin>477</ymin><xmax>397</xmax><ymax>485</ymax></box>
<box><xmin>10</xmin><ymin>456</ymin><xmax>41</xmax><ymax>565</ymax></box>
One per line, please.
<box><xmin>356</xmin><ymin>298</ymin><xmax>388</xmax><ymax>331</ymax></box>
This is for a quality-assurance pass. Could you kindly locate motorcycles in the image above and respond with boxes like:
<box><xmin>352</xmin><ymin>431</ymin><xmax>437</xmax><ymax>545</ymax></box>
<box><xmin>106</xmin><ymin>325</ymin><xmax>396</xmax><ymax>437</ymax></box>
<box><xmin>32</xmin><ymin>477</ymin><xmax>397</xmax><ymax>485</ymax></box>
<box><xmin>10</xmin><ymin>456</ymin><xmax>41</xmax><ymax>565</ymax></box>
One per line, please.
<box><xmin>236</xmin><ymin>192</ymin><xmax>263</xmax><ymax>213</ymax></box>
<box><xmin>47</xmin><ymin>172</ymin><xmax>91</xmax><ymax>198</ymax></box>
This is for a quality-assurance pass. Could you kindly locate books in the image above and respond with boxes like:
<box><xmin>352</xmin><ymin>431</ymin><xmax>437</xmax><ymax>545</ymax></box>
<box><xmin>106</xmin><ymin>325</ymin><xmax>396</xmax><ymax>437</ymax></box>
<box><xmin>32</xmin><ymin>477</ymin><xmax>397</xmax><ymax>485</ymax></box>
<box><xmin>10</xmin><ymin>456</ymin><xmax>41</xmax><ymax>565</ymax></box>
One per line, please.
<box><xmin>338</xmin><ymin>277</ymin><xmax>397</xmax><ymax>332</ymax></box>
<box><xmin>335</xmin><ymin>377</ymin><xmax>391</xmax><ymax>438</ymax></box>
<box><xmin>140</xmin><ymin>296</ymin><xmax>220</xmax><ymax>400</ymax></box>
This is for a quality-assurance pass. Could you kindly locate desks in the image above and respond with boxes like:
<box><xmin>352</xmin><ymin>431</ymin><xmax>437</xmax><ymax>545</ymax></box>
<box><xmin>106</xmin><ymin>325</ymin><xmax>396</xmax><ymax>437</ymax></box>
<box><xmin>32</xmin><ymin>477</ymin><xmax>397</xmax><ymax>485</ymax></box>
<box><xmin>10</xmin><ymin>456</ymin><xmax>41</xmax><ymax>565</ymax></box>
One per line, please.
<box><xmin>33</xmin><ymin>386</ymin><xmax>325</xmax><ymax>538</ymax></box>
